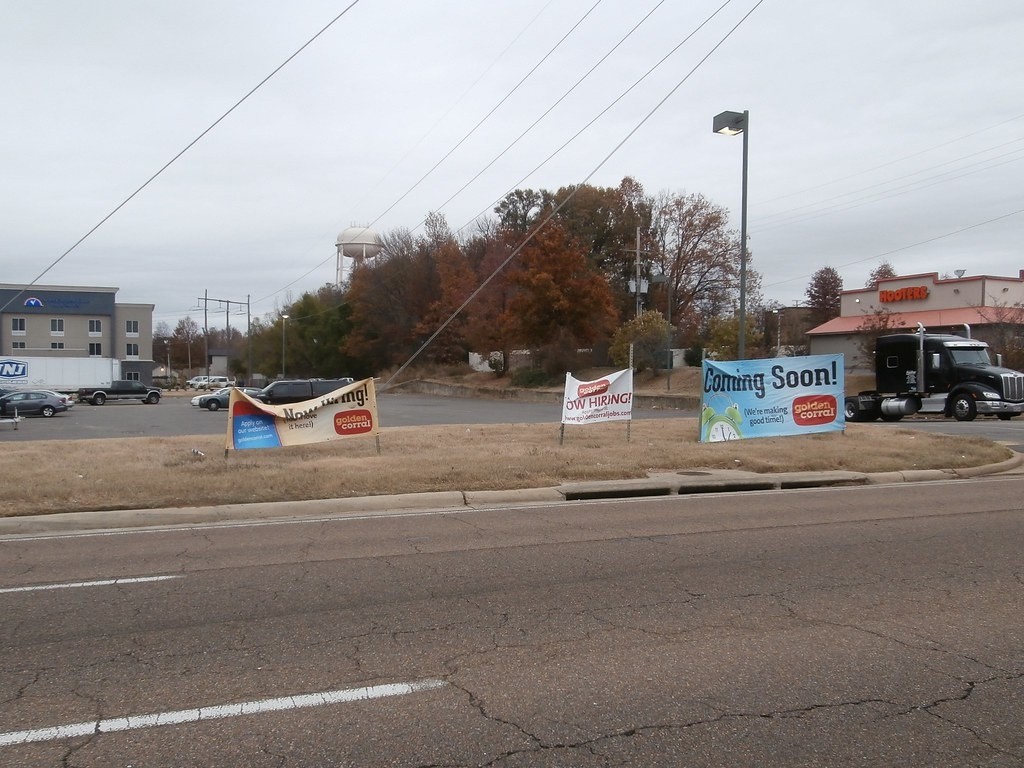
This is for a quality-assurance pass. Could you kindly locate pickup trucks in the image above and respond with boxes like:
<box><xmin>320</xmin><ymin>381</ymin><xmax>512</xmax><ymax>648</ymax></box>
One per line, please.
<box><xmin>257</xmin><ymin>379</ymin><xmax>350</xmax><ymax>405</ymax></box>
<box><xmin>204</xmin><ymin>377</ymin><xmax>235</xmax><ymax>388</ymax></box>
<box><xmin>78</xmin><ymin>380</ymin><xmax>163</xmax><ymax>406</ymax></box>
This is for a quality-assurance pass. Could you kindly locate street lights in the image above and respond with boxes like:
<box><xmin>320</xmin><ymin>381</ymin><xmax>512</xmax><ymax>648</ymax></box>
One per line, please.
<box><xmin>713</xmin><ymin>110</ymin><xmax>749</xmax><ymax>360</ymax></box>
<box><xmin>773</xmin><ymin>310</ymin><xmax>780</xmax><ymax>348</ymax></box>
<box><xmin>161</xmin><ymin>367</ymin><xmax>167</xmax><ymax>384</ymax></box>
<box><xmin>282</xmin><ymin>315</ymin><xmax>289</xmax><ymax>381</ymax></box>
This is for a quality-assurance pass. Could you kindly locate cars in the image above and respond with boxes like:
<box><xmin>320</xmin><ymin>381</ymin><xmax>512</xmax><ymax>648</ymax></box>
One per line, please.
<box><xmin>8</xmin><ymin>390</ymin><xmax>75</xmax><ymax>407</ymax></box>
<box><xmin>191</xmin><ymin>388</ymin><xmax>228</xmax><ymax>406</ymax></box>
<box><xmin>0</xmin><ymin>391</ymin><xmax>67</xmax><ymax>417</ymax></box>
<box><xmin>199</xmin><ymin>387</ymin><xmax>262</xmax><ymax>411</ymax></box>
<box><xmin>186</xmin><ymin>376</ymin><xmax>213</xmax><ymax>389</ymax></box>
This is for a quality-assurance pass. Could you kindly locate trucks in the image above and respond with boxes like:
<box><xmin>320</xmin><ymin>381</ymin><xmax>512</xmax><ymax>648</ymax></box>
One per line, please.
<box><xmin>844</xmin><ymin>322</ymin><xmax>1024</xmax><ymax>422</ymax></box>
